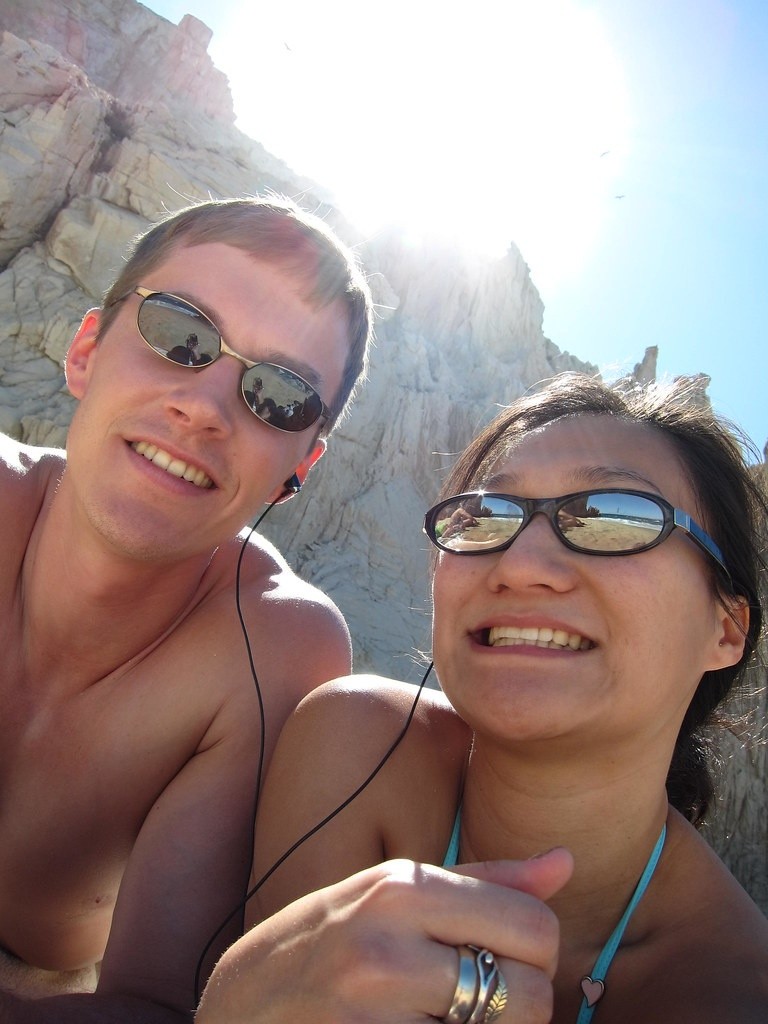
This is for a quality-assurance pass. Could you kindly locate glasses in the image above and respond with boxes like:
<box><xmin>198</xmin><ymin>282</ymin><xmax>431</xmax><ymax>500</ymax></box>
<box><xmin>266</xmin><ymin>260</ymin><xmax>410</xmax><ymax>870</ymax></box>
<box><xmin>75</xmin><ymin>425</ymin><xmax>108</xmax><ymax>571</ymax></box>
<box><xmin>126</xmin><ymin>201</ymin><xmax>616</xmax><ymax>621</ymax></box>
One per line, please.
<box><xmin>107</xmin><ymin>283</ymin><xmax>332</xmax><ymax>434</ymax></box>
<box><xmin>421</xmin><ymin>488</ymin><xmax>734</xmax><ymax>584</ymax></box>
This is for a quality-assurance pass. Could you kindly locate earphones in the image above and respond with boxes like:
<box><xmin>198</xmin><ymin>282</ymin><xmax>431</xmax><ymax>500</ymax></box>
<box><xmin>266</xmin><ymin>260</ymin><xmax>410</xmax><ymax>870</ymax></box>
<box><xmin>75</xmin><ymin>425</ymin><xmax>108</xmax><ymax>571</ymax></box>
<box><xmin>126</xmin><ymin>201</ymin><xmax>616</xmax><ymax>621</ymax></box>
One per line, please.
<box><xmin>283</xmin><ymin>472</ymin><xmax>301</xmax><ymax>495</ymax></box>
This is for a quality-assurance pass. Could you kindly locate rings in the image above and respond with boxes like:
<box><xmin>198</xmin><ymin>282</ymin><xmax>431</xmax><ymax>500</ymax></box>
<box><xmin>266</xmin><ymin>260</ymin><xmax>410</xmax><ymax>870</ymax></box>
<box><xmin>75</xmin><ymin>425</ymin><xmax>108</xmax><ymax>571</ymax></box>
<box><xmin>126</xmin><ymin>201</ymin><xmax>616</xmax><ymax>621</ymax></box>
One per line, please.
<box><xmin>445</xmin><ymin>944</ymin><xmax>507</xmax><ymax>1024</ymax></box>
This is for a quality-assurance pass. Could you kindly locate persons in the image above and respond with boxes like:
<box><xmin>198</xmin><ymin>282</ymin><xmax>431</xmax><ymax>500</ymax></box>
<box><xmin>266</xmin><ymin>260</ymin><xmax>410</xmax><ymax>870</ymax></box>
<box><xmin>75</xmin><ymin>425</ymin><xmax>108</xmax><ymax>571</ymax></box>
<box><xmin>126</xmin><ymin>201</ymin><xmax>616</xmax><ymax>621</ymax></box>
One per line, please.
<box><xmin>166</xmin><ymin>333</ymin><xmax>212</xmax><ymax>367</ymax></box>
<box><xmin>254</xmin><ymin>385</ymin><xmax>303</xmax><ymax>430</ymax></box>
<box><xmin>435</xmin><ymin>503</ymin><xmax>477</xmax><ymax>538</ymax></box>
<box><xmin>192</xmin><ymin>380</ymin><xmax>768</xmax><ymax>1024</ymax></box>
<box><xmin>558</xmin><ymin>507</ymin><xmax>581</xmax><ymax>528</ymax></box>
<box><xmin>0</xmin><ymin>195</ymin><xmax>371</xmax><ymax>1024</ymax></box>
